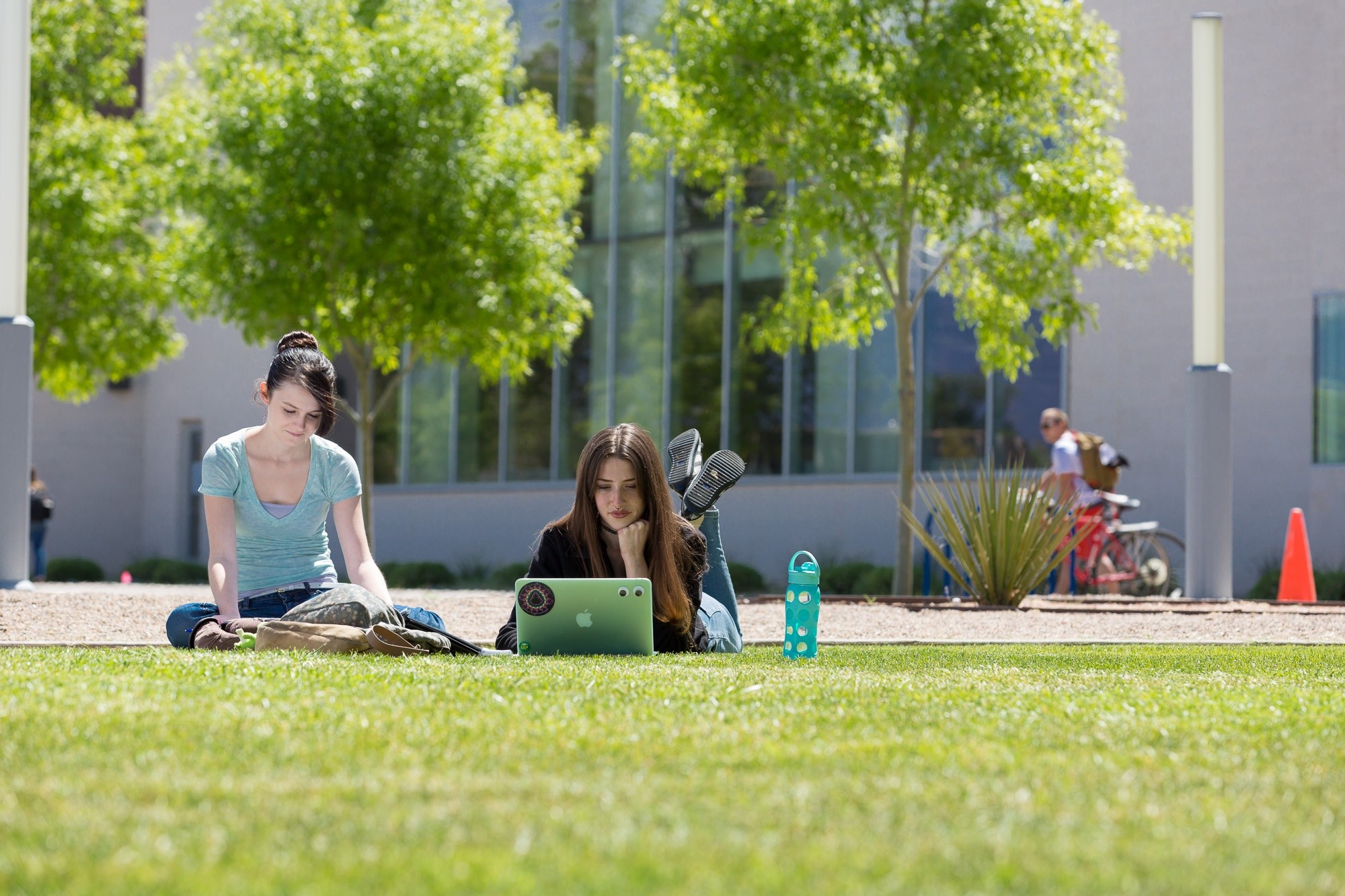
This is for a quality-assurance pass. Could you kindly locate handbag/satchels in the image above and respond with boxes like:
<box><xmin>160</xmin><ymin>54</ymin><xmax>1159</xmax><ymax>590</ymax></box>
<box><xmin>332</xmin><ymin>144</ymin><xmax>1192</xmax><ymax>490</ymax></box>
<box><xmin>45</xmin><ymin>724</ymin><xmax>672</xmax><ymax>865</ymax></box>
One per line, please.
<box><xmin>254</xmin><ymin>583</ymin><xmax>451</xmax><ymax>657</ymax></box>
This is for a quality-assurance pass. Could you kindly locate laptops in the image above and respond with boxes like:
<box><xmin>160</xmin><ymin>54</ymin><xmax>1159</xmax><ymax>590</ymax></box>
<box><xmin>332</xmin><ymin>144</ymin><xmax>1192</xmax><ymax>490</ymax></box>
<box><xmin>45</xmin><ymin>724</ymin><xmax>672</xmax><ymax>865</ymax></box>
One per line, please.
<box><xmin>516</xmin><ymin>578</ymin><xmax>658</xmax><ymax>656</ymax></box>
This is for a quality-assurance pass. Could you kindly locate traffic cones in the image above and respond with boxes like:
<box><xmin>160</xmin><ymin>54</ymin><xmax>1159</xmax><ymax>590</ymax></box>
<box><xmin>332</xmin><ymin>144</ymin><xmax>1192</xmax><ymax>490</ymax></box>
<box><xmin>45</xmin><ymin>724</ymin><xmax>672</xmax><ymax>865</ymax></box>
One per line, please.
<box><xmin>1277</xmin><ymin>508</ymin><xmax>1318</xmax><ymax>604</ymax></box>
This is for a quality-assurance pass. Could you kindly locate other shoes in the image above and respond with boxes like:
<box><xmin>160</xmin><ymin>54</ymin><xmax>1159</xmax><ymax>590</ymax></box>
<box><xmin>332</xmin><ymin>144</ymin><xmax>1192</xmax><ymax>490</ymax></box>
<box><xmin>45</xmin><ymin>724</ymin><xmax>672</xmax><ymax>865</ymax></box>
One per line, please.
<box><xmin>681</xmin><ymin>449</ymin><xmax>745</xmax><ymax>520</ymax></box>
<box><xmin>667</xmin><ymin>429</ymin><xmax>703</xmax><ymax>497</ymax></box>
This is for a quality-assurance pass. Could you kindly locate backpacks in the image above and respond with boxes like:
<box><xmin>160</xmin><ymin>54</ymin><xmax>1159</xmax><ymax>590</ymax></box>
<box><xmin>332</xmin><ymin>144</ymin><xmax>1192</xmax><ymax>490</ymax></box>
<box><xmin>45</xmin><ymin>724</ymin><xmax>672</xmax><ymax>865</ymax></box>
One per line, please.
<box><xmin>1075</xmin><ymin>431</ymin><xmax>1129</xmax><ymax>492</ymax></box>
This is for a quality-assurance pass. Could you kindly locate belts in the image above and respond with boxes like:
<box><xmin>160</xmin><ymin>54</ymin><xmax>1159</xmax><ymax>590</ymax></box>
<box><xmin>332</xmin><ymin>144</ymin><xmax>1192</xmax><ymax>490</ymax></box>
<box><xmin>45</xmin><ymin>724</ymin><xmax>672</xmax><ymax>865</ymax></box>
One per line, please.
<box><xmin>236</xmin><ymin>580</ymin><xmax>338</xmax><ymax>606</ymax></box>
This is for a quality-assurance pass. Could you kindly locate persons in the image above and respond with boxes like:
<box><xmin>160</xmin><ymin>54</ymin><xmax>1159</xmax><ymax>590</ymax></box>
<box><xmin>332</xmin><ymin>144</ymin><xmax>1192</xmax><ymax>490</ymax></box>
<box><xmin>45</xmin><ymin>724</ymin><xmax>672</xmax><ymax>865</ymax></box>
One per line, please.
<box><xmin>166</xmin><ymin>331</ymin><xmax>445</xmax><ymax>649</ymax></box>
<box><xmin>30</xmin><ymin>467</ymin><xmax>54</xmax><ymax>582</ymax></box>
<box><xmin>495</xmin><ymin>423</ymin><xmax>745</xmax><ymax>655</ymax></box>
<box><xmin>1031</xmin><ymin>408</ymin><xmax>1122</xmax><ymax>596</ymax></box>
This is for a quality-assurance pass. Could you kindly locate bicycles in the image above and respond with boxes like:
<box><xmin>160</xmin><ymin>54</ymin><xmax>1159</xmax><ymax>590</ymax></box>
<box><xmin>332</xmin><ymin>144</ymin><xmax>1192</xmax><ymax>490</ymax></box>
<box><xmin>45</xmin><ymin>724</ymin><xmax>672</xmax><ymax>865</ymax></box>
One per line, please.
<box><xmin>1030</xmin><ymin>489</ymin><xmax>1186</xmax><ymax>599</ymax></box>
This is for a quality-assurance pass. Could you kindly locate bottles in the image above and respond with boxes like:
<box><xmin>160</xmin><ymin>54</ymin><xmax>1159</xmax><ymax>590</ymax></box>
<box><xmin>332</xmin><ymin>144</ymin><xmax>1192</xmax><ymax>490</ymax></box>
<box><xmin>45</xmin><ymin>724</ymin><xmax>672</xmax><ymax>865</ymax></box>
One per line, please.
<box><xmin>783</xmin><ymin>550</ymin><xmax>821</xmax><ymax>661</ymax></box>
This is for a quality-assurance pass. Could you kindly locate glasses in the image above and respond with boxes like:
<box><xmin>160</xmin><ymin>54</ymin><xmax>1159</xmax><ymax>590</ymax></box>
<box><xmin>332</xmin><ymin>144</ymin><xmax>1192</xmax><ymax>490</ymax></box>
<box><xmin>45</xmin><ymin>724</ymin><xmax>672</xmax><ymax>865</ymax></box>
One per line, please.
<box><xmin>1043</xmin><ymin>419</ymin><xmax>1061</xmax><ymax>431</ymax></box>
